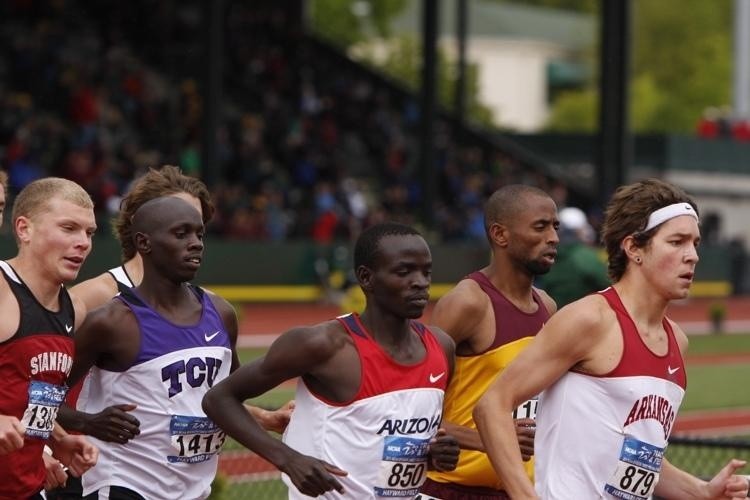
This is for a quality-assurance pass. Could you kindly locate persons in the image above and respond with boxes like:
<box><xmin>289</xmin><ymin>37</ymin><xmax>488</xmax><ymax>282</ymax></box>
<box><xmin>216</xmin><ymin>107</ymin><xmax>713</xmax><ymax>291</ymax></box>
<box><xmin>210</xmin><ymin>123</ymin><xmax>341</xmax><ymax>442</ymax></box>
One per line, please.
<box><xmin>64</xmin><ymin>177</ymin><xmax>97</xmax><ymax>487</ymax></box>
<box><xmin>415</xmin><ymin>184</ymin><xmax>562</xmax><ymax>500</ymax></box>
<box><xmin>473</xmin><ymin>178</ymin><xmax>750</xmax><ymax>500</ymax></box>
<box><xmin>68</xmin><ymin>169</ymin><xmax>297</xmax><ymax>500</ymax></box>
<box><xmin>56</xmin><ymin>196</ymin><xmax>241</xmax><ymax>500</ymax></box>
<box><xmin>202</xmin><ymin>223</ymin><xmax>459</xmax><ymax>499</ymax></box>
